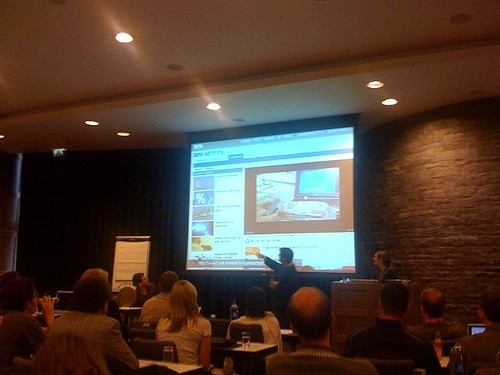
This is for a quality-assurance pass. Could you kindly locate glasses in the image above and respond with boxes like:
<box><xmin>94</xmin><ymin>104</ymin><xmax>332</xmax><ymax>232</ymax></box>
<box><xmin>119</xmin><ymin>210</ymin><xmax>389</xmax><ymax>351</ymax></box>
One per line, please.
<box><xmin>32</xmin><ymin>293</ymin><xmax>39</xmax><ymax>298</ymax></box>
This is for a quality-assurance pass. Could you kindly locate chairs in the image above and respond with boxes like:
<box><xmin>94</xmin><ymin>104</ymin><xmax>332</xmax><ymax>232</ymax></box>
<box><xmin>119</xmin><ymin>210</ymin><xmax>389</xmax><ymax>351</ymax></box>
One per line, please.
<box><xmin>43</xmin><ymin>291</ymin><xmax>426</xmax><ymax>375</ymax></box>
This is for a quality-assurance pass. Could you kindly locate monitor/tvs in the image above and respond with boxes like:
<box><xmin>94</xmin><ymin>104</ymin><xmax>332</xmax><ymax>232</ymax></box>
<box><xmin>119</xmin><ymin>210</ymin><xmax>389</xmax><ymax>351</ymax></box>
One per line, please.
<box><xmin>467</xmin><ymin>323</ymin><xmax>487</xmax><ymax>336</ymax></box>
<box><xmin>53</xmin><ymin>290</ymin><xmax>74</xmax><ymax>310</ymax></box>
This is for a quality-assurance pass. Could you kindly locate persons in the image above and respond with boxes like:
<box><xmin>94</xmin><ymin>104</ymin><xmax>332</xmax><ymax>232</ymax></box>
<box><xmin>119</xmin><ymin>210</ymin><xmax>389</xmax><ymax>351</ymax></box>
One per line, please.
<box><xmin>50</xmin><ymin>277</ymin><xmax>140</xmax><ymax>372</ymax></box>
<box><xmin>80</xmin><ymin>269</ymin><xmax>129</xmax><ymax>341</ymax></box>
<box><xmin>258</xmin><ymin>246</ymin><xmax>298</xmax><ymax>308</ymax></box>
<box><xmin>449</xmin><ymin>287</ymin><xmax>500</xmax><ymax>375</ymax></box>
<box><xmin>226</xmin><ymin>286</ymin><xmax>282</xmax><ymax>365</ymax></box>
<box><xmin>371</xmin><ymin>251</ymin><xmax>400</xmax><ymax>283</ymax></box>
<box><xmin>343</xmin><ymin>281</ymin><xmax>445</xmax><ymax>375</ymax></box>
<box><xmin>132</xmin><ymin>273</ymin><xmax>159</xmax><ymax>308</ymax></box>
<box><xmin>407</xmin><ymin>287</ymin><xmax>464</xmax><ymax>356</ymax></box>
<box><xmin>141</xmin><ymin>271</ymin><xmax>179</xmax><ymax>325</ymax></box>
<box><xmin>0</xmin><ymin>271</ymin><xmax>56</xmax><ymax>375</ymax></box>
<box><xmin>29</xmin><ymin>331</ymin><xmax>112</xmax><ymax>375</ymax></box>
<box><xmin>155</xmin><ymin>278</ymin><xmax>212</xmax><ymax>372</ymax></box>
<box><xmin>267</xmin><ymin>288</ymin><xmax>380</xmax><ymax>375</ymax></box>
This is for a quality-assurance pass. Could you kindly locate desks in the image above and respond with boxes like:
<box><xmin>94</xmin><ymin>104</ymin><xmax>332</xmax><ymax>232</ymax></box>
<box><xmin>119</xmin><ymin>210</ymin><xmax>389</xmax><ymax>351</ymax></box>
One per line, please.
<box><xmin>214</xmin><ymin>342</ymin><xmax>278</xmax><ymax>375</ymax></box>
<box><xmin>138</xmin><ymin>359</ymin><xmax>203</xmax><ymax>375</ymax></box>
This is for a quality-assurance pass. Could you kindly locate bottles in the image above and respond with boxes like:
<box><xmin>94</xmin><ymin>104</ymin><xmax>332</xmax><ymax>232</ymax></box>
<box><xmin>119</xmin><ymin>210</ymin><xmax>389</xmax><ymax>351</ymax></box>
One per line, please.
<box><xmin>230</xmin><ymin>297</ymin><xmax>238</xmax><ymax>321</ymax></box>
